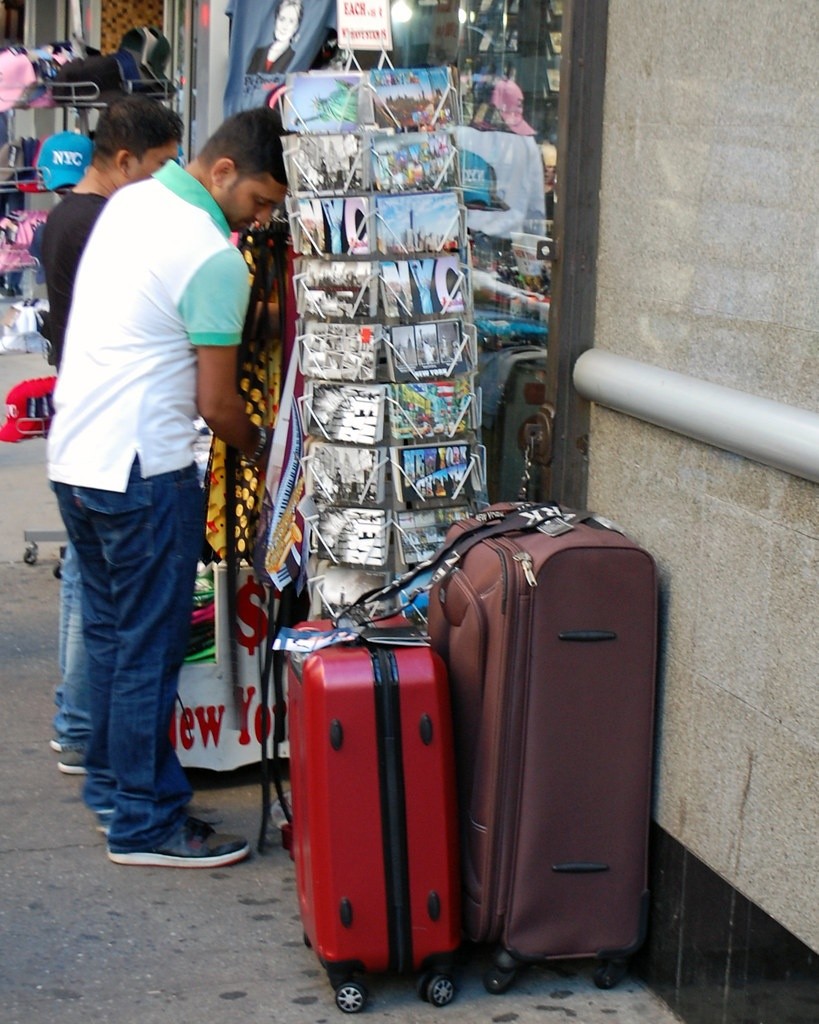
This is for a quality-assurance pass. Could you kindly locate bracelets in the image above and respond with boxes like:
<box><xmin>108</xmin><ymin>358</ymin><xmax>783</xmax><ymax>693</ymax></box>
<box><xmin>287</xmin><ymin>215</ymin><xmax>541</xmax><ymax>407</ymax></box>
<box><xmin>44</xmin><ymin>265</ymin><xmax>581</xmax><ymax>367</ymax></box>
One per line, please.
<box><xmin>240</xmin><ymin>427</ymin><xmax>268</xmax><ymax>468</ymax></box>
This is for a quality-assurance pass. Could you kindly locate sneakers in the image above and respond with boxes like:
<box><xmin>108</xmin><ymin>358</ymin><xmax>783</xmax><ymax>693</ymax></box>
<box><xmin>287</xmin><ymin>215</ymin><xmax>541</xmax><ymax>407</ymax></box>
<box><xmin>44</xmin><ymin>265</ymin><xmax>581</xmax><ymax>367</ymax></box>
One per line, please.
<box><xmin>107</xmin><ymin>815</ymin><xmax>250</xmax><ymax>869</ymax></box>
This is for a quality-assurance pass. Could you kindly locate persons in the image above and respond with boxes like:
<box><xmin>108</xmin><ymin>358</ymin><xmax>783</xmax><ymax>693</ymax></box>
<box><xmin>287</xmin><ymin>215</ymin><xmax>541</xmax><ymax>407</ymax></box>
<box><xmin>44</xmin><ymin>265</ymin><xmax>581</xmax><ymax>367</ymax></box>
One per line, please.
<box><xmin>39</xmin><ymin>92</ymin><xmax>289</xmax><ymax>873</ymax></box>
<box><xmin>246</xmin><ymin>0</ymin><xmax>304</xmax><ymax>74</ymax></box>
<box><xmin>473</xmin><ymin>61</ymin><xmax>517</xmax><ymax>123</ymax></box>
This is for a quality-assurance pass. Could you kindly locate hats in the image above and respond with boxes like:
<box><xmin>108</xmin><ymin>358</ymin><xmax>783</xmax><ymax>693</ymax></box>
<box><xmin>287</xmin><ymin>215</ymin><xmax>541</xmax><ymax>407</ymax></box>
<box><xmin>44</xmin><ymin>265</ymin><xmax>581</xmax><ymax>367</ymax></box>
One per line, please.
<box><xmin>0</xmin><ymin>132</ymin><xmax>95</xmax><ymax>190</ymax></box>
<box><xmin>0</xmin><ymin>27</ymin><xmax>180</xmax><ymax>112</ymax></box>
<box><xmin>468</xmin><ymin>73</ymin><xmax>539</xmax><ymax>138</ymax></box>
<box><xmin>458</xmin><ymin>150</ymin><xmax>510</xmax><ymax>215</ymax></box>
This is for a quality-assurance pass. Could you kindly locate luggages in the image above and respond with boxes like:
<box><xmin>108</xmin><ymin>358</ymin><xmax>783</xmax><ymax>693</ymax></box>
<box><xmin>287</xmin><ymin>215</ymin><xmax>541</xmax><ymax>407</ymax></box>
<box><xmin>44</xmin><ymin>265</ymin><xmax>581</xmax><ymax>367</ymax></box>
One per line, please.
<box><xmin>426</xmin><ymin>500</ymin><xmax>655</xmax><ymax>992</ymax></box>
<box><xmin>289</xmin><ymin>615</ymin><xmax>463</xmax><ymax>1012</ymax></box>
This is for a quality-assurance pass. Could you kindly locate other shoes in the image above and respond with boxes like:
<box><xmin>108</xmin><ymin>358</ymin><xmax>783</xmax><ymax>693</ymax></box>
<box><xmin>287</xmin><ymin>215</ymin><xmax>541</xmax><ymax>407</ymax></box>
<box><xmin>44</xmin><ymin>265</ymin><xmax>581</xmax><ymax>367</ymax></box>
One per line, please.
<box><xmin>58</xmin><ymin>749</ymin><xmax>90</xmax><ymax>775</ymax></box>
